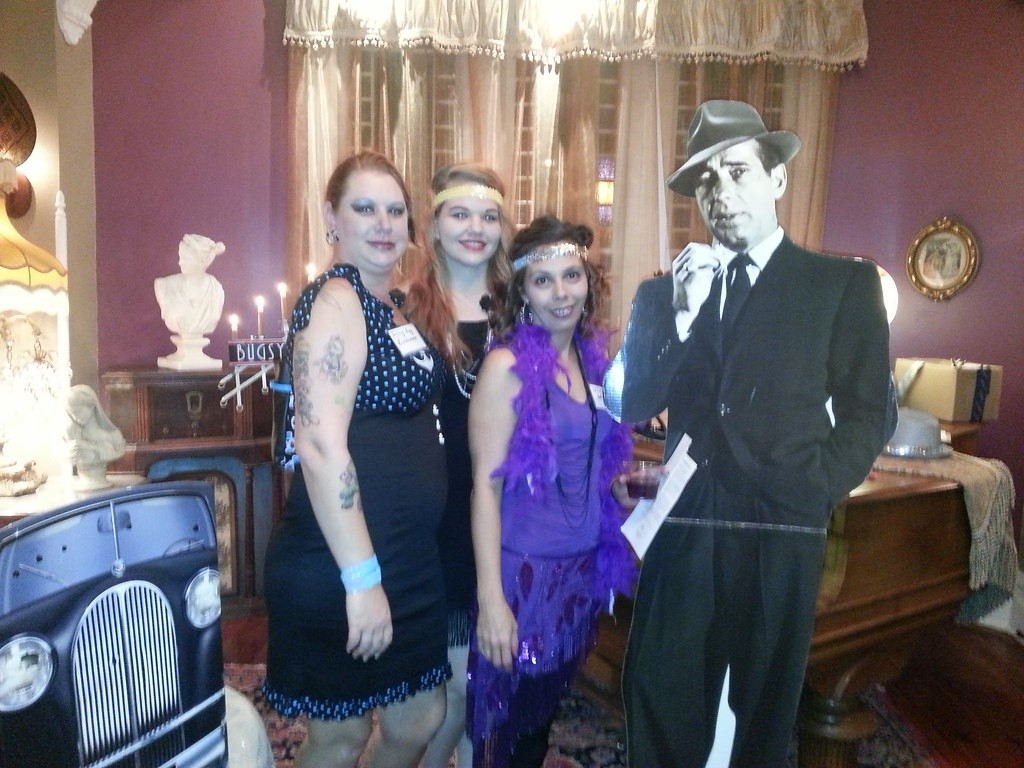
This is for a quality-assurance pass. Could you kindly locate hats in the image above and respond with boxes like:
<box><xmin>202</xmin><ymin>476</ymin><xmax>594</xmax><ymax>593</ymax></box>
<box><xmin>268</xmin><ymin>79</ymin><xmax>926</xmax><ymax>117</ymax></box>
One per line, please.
<box><xmin>665</xmin><ymin>99</ymin><xmax>800</xmax><ymax>196</ymax></box>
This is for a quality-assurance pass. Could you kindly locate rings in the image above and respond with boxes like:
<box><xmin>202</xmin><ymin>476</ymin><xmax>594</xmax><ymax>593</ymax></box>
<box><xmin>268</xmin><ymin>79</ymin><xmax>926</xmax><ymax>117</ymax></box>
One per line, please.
<box><xmin>481</xmin><ymin>648</ymin><xmax>491</xmax><ymax>655</ymax></box>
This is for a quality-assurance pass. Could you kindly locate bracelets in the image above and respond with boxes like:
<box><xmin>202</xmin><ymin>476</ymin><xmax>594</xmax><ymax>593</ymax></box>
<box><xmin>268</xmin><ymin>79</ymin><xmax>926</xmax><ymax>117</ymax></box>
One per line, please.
<box><xmin>340</xmin><ymin>556</ymin><xmax>381</xmax><ymax>591</ymax></box>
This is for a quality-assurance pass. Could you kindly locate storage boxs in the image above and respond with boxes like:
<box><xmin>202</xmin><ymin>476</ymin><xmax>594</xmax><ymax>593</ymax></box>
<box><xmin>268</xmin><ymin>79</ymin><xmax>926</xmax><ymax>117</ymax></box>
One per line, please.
<box><xmin>894</xmin><ymin>358</ymin><xmax>1004</xmax><ymax>424</ymax></box>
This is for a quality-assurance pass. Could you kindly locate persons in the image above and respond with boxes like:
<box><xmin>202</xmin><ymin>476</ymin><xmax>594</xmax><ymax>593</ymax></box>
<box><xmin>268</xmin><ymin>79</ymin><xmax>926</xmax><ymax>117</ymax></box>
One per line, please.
<box><xmin>604</xmin><ymin>100</ymin><xmax>898</xmax><ymax>768</ymax></box>
<box><xmin>154</xmin><ymin>235</ymin><xmax>224</xmax><ymax>334</ymax></box>
<box><xmin>264</xmin><ymin>152</ymin><xmax>453</xmax><ymax>768</ymax></box>
<box><xmin>391</xmin><ymin>162</ymin><xmax>520</xmax><ymax>768</ymax></box>
<box><xmin>62</xmin><ymin>384</ymin><xmax>126</xmax><ymax>465</ymax></box>
<box><xmin>468</xmin><ymin>217</ymin><xmax>670</xmax><ymax>768</ymax></box>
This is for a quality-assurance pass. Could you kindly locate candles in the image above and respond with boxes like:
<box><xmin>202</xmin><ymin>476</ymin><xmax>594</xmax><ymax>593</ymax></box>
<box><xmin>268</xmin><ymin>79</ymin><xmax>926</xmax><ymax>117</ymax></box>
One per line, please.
<box><xmin>54</xmin><ymin>187</ymin><xmax>70</xmax><ymax>272</ymax></box>
<box><xmin>279</xmin><ymin>283</ymin><xmax>288</xmax><ymax>320</ymax></box>
<box><xmin>230</xmin><ymin>313</ymin><xmax>240</xmax><ymax>341</ymax></box>
<box><xmin>257</xmin><ymin>295</ymin><xmax>265</xmax><ymax>336</ymax></box>
<box><xmin>307</xmin><ymin>262</ymin><xmax>315</xmax><ymax>284</ymax></box>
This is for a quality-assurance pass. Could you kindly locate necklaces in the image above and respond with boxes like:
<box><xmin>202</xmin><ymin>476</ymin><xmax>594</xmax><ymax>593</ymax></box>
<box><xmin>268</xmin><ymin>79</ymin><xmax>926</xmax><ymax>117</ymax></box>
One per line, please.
<box><xmin>451</xmin><ymin>326</ymin><xmax>495</xmax><ymax>398</ymax></box>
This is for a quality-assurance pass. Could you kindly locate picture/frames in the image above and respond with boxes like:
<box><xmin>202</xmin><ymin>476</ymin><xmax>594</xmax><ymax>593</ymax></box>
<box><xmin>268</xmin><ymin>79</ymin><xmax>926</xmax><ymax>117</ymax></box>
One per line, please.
<box><xmin>903</xmin><ymin>216</ymin><xmax>984</xmax><ymax>302</ymax></box>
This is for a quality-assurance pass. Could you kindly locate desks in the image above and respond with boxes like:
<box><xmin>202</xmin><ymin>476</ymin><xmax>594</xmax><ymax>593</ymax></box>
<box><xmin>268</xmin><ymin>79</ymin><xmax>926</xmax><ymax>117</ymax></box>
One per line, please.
<box><xmin>583</xmin><ymin>440</ymin><xmax>1012</xmax><ymax>768</ymax></box>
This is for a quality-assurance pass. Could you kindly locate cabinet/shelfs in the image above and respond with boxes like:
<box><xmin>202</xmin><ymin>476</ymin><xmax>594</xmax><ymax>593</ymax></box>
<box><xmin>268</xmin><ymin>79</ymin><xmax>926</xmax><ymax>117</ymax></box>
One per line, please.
<box><xmin>98</xmin><ymin>367</ymin><xmax>276</xmax><ymax>618</ymax></box>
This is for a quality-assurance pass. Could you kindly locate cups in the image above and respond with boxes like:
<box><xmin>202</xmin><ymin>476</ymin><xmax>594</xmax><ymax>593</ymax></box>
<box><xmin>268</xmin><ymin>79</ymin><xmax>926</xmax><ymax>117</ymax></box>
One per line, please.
<box><xmin>622</xmin><ymin>460</ymin><xmax>664</xmax><ymax>499</ymax></box>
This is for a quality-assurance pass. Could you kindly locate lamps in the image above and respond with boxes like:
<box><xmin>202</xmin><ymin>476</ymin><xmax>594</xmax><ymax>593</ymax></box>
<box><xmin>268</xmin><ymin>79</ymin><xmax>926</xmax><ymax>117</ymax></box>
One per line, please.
<box><xmin>0</xmin><ymin>158</ymin><xmax>72</xmax><ymax>499</ymax></box>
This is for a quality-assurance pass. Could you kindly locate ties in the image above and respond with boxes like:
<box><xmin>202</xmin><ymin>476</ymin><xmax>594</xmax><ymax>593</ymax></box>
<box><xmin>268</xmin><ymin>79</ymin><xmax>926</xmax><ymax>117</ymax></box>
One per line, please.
<box><xmin>718</xmin><ymin>254</ymin><xmax>754</xmax><ymax>363</ymax></box>
